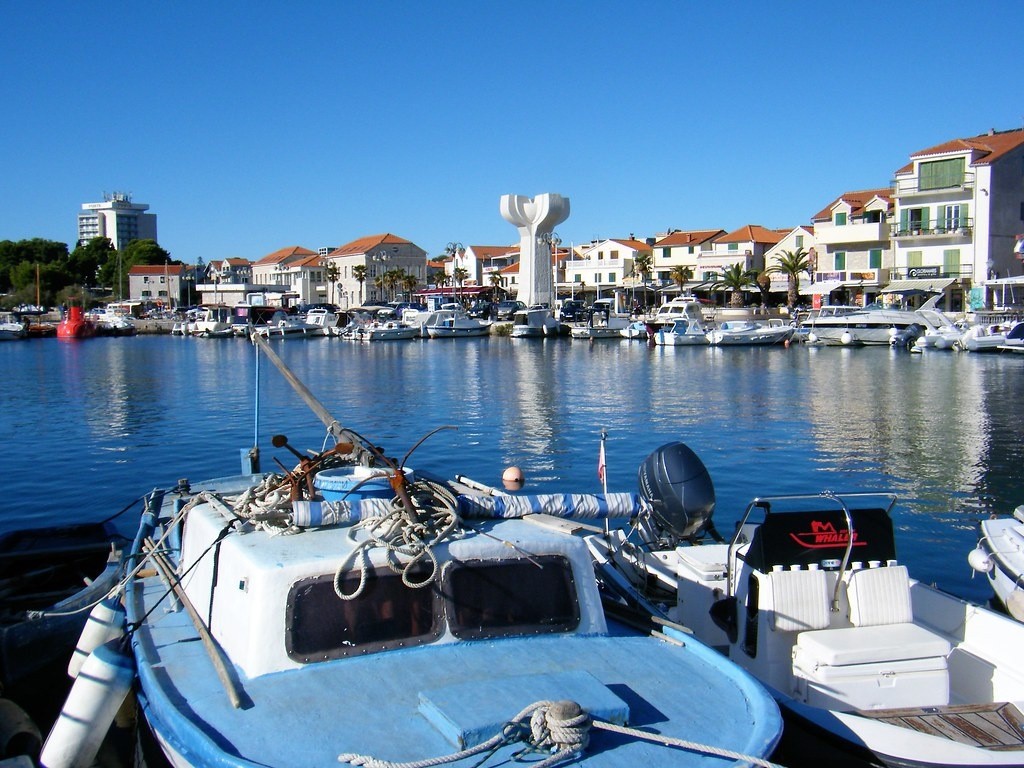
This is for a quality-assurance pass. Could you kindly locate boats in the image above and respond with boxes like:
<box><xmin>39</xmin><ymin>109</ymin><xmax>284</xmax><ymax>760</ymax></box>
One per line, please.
<box><xmin>0</xmin><ymin>293</ymin><xmax>1024</xmax><ymax>354</ymax></box>
<box><xmin>123</xmin><ymin>322</ymin><xmax>787</xmax><ymax>768</ymax></box>
<box><xmin>966</xmin><ymin>505</ymin><xmax>1024</xmax><ymax>624</ymax></box>
<box><xmin>580</xmin><ymin>437</ymin><xmax>1024</xmax><ymax>768</ymax></box>
<box><xmin>0</xmin><ymin>517</ymin><xmax>129</xmax><ymax>651</ymax></box>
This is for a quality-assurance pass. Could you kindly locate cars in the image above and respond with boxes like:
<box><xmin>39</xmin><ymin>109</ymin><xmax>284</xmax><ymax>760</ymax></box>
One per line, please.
<box><xmin>559</xmin><ymin>299</ymin><xmax>590</xmax><ymax>322</ymax></box>
<box><xmin>298</xmin><ymin>302</ymin><xmax>340</xmax><ymax>314</ymax></box>
<box><xmin>441</xmin><ymin>299</ymin><xmax>548</xmax><ymax>321</ymax></box>
<box><xmin>352</xmin><ymin>298</ymin><xmax>427</xmax><ymax>321</ymax></box>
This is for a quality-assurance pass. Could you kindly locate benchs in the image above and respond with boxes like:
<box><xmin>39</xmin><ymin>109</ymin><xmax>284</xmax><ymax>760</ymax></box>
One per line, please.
<box><xmin>762</xmin><ymin>565</ymin><xmax>949</xmax><ymax>709</ymax></box>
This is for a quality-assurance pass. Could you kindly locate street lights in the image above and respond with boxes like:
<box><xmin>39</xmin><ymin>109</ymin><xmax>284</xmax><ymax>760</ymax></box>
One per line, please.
<box><xmin>372</xmin><ymin>249</ymin><xmax>392</xmax><ymax>302</ymax></box>
<box><xmin>181</xmin><ymin>273</ymin><xmax>195</xmax><ymax>308</ymax></box>
<box><xmin>144</xmin><ymin>280</ymin><xmax>154</xmax><ymax>296</ymax></box>
<box><xmin>237</xmin><ymin>266</ymin><xmax>252</xmax><ymax>300</ymax></box>
<box><xmin>317</xmin><ymin>255</ymin><xmax>341</xmax><ymax>307</ymax></box>
<box><xmin>444</xmin><ymin>241</ymin><xmax>466</xmax><ymax>303</ymax></box>
<box><xmin>274</xmin><ymin>262</ymin><xmax>289</xmax><ymax>285</ymax></box>
<box><xmin>537</xmin><ymin>232</ymin><xmax>562</xmax><ymax>311</ymax></box>
<box><xmin>207</xmin><ymin>267</ymin><xmax>221</xmax><ymax>304</ymax></box>
